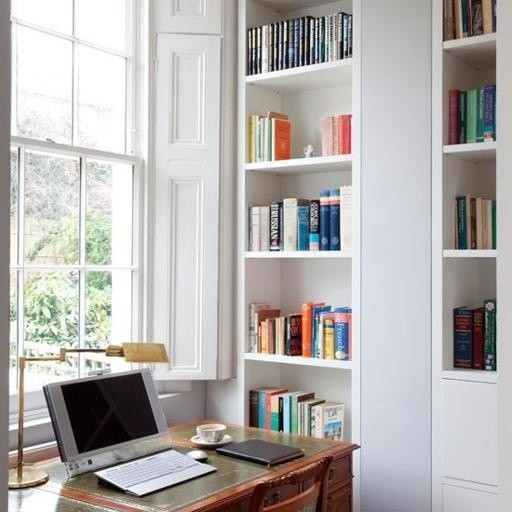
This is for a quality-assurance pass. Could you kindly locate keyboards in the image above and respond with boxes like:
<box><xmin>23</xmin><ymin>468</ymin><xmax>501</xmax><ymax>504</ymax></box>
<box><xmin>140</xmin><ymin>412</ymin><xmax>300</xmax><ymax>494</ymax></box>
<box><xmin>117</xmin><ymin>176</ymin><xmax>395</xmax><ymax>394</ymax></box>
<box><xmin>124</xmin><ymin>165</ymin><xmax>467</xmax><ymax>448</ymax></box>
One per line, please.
<box><xmin>95</xmin><ymin>450</ymin><xmax>219</xmax><ymax>499</ymax></box>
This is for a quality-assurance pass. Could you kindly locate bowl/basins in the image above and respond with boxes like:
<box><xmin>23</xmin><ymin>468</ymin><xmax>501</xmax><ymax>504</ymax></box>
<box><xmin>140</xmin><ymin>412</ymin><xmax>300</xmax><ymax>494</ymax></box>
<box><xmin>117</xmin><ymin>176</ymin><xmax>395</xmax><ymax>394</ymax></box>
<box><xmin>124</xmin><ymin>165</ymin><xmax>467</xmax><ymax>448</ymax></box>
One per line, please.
<box><xmin>194</xmin><ymin>423</ymin><xmax>228</xmax><ymax>443</ymax></box>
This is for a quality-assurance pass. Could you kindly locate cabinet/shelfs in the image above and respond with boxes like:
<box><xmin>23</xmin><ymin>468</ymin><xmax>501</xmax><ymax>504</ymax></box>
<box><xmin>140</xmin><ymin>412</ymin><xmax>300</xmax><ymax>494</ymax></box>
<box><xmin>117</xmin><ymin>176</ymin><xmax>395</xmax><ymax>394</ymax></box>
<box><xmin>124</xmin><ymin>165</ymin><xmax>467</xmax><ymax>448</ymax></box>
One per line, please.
<box><xmin>435</xmin><ymin>1</ymin><xmax>512</xmax><ymax>509</ymax></box>
<box><xmin>234</xmin><ymin>0</ymin><xmax>360</xmax><ymax>445</ymax></box>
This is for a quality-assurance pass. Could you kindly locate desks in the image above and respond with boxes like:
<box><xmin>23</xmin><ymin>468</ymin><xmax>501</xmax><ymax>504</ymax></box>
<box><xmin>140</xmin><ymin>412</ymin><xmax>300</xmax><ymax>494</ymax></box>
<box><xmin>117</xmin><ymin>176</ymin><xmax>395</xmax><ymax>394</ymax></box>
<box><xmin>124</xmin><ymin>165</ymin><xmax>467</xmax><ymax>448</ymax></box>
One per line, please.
<box><xmin>8</xmin><ymin>416</ymin><xmax>361</xmax><ymax>512</ymax></box>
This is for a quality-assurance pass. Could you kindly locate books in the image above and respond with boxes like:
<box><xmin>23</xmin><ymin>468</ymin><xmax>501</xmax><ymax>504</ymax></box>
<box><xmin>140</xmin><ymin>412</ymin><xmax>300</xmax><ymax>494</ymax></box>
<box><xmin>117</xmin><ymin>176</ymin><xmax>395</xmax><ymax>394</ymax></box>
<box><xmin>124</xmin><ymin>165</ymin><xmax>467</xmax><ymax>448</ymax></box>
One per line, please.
<box><xmin>321</xmin><ymin>113</ymin><xmax>352</xmax><ymax>157</ymax></box>
<box><xmin>452</xmin><ymin>299</ymin><xmax>497</xmax><ymax>371</ymax></box>
<box><xmin>216</xmin><ymin>438</ymin><xmax>305</xmax><ymax>469</ymax></box>
<box><xmin>448</xmin><ymin>85</ymin><xmax>496</xmax><ymax>146</ymax></box>
<box><xmin>246</xmin><ymin>111</ymin><xmax>291</xmax><ymax>164</ymax></box>
<box><xmin>454</xmin><ymin>196</ymin><xmax>496</xmax><ymax>250</ymax></box>
<box><xmin>440</xmin><ymin>0</ymin><xmax>499</xmax><ymax>42</ymax></box>
<box><xmin>246</xmin><ymin>12</ymin><xmax>353</xmax><ymax>76</ymax></box>
<box><xmin>249</xmin><ymin>385</ymin><xmax>344</xmax><ymax>442</ymax></box>
<box><xmin>248</xmin><ymin>184</ymin><xmax>353</xmax><ymax>251</ymax></box>
<box><xmin>248</xmin><ymin>299</ymin><xmax>352</xmax><ymax>361</ymax></box>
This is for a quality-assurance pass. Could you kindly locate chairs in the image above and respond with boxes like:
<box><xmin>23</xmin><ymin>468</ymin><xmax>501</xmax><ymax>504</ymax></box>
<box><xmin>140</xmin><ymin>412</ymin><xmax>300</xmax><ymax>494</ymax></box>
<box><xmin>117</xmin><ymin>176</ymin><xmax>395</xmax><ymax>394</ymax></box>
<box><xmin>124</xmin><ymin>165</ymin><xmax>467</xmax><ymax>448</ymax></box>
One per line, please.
<box><xmin>250</xmin><ymin>452</ymin><xmax>336</xmax><ymax>510</ymax></box>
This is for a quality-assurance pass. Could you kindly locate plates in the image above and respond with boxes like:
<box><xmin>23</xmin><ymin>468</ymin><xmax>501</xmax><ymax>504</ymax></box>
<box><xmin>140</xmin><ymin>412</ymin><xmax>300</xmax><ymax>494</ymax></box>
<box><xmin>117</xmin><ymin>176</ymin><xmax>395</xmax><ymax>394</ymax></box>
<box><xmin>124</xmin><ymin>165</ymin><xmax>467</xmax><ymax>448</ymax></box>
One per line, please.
<box><xmin>190</xmin><ymin>433</ymin><xmax>233</xmax><ymax>446</ymax></box>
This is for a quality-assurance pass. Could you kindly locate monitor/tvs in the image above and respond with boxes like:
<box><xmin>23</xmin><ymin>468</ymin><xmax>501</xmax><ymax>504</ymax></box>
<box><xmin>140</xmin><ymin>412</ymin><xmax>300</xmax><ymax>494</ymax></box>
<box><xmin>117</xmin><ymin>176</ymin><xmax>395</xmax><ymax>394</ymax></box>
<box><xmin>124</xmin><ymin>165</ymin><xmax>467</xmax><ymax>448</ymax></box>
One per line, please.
<box><xmin>43</xmin><ymin>367</ymin><xmax>173</xmax><ymax>476</ymax></box>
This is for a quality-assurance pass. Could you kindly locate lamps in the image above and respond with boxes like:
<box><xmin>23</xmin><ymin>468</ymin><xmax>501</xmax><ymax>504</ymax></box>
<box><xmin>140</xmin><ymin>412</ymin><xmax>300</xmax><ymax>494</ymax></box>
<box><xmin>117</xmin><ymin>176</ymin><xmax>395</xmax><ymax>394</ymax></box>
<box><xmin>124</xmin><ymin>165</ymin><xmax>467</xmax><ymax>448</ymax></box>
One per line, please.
<box><xmin>8</xmin><ymin>340</ymin><xmax>170</xmax><ymax>490</ymax></box>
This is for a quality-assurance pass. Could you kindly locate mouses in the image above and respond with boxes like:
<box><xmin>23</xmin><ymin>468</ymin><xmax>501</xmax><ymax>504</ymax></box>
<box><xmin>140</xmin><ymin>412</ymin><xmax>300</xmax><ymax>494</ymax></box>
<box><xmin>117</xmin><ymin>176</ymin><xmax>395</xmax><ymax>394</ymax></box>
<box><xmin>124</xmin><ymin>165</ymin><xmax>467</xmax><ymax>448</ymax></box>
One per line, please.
<box><xmin>187</xmin><ymin>451</ymin><xmax>207</xmax><ymax>462</ymax></box>
